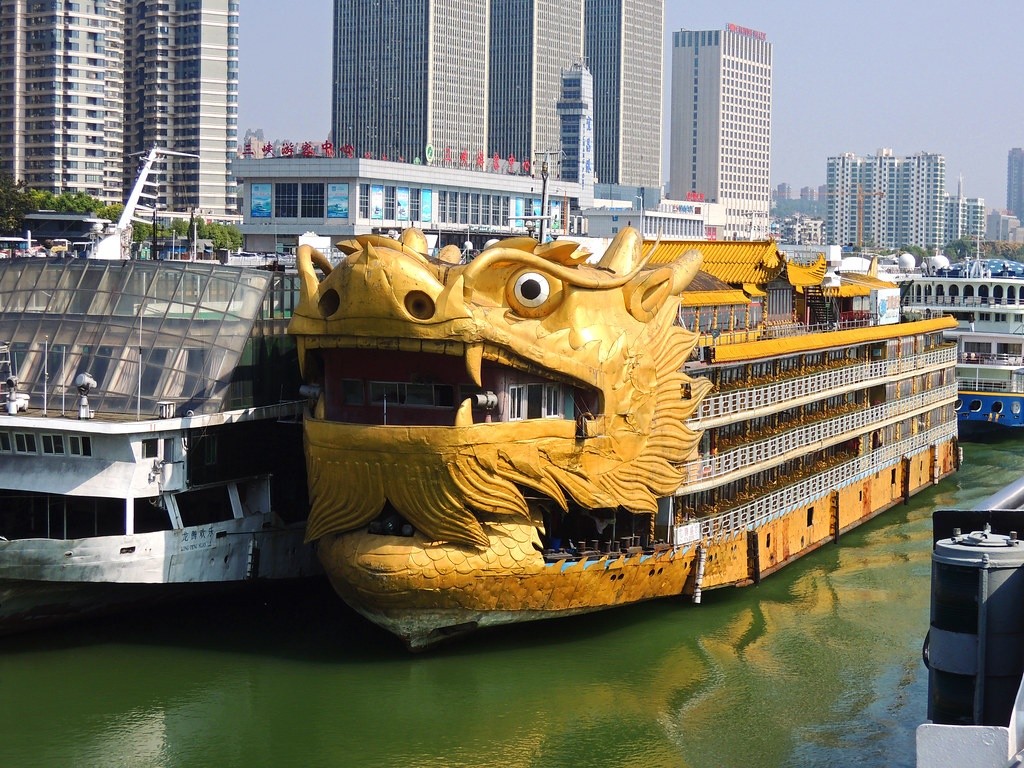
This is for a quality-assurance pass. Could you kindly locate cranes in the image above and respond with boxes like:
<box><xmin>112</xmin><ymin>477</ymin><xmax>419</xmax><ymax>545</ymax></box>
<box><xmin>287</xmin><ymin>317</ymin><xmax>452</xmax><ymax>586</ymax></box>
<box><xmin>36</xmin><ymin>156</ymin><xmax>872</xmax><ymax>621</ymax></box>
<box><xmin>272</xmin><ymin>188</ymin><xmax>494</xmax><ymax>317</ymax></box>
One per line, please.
<box><xmin>768</xmin><ymin>183</ymin><xmax>886</xmax><ymax>245</ymax></box>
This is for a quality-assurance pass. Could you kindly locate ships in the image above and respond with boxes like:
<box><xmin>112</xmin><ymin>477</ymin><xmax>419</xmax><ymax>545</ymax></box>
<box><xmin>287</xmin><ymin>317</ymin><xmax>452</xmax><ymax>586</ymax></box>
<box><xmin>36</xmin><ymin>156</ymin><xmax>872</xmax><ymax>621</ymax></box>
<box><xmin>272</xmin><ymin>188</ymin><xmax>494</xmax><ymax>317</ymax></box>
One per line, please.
<box><xmin>896</xmin><ymin>199</ymin><xmax>1024</xmax><ymax>432</ymax></box>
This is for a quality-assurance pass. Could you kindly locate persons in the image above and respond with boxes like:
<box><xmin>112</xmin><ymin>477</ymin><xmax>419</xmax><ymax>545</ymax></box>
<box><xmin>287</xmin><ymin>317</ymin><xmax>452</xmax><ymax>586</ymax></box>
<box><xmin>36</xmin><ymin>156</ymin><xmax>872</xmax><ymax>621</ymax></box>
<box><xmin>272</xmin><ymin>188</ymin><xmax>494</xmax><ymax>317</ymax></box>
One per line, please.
<box><xmin>926</xmin><ymin>309</ymin><xmax>931</xmax><ymax>319</ymax></box>
<box><xmin>968</xmin><ymin>313</ymin><xmax>975</xmax><ymax>333</ymax></box>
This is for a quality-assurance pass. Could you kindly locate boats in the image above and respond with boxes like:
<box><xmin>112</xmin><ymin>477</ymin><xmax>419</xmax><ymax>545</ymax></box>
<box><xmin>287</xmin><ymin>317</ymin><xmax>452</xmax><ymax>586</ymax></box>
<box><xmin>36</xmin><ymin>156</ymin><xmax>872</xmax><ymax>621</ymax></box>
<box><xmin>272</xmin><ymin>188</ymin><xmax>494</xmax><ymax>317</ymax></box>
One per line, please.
<box><xmin>281</xmin><ymin>157</ymin><xmax>964</xmax><ymax>650</ymax></box>
<box><xmin>0</xmin><ymin>143</ymin><xmax>342</xmax><ymax>617</ymax></box>
<box><xmin>915</xmin><ymin>468</ymin><xmax>1024</xmax><ymax>768</ymax></box>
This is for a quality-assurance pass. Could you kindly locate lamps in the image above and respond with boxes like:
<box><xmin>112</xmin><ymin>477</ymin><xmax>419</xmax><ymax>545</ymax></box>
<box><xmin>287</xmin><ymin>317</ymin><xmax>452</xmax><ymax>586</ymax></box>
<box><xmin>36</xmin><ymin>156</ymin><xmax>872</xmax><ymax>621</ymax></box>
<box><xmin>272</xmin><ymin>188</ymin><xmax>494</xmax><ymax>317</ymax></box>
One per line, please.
<box><xmin>6</xmin><ymin>376</ymin><xmax>19</xmax><ymax>388</ymax></box>
<box><xmin>76</xmin><ymin>372</ymin><xmax>97</xmax><ymax>392</ymax></box>
<box><xmin>299</xmin><ymin>384</ymin><xmax>321</xmax><ymax>408</ymax></box>
<box><xmin>472</xmin><ymin>391</ymin><xmax>498</xmax><ymax>415</ymax></box>
